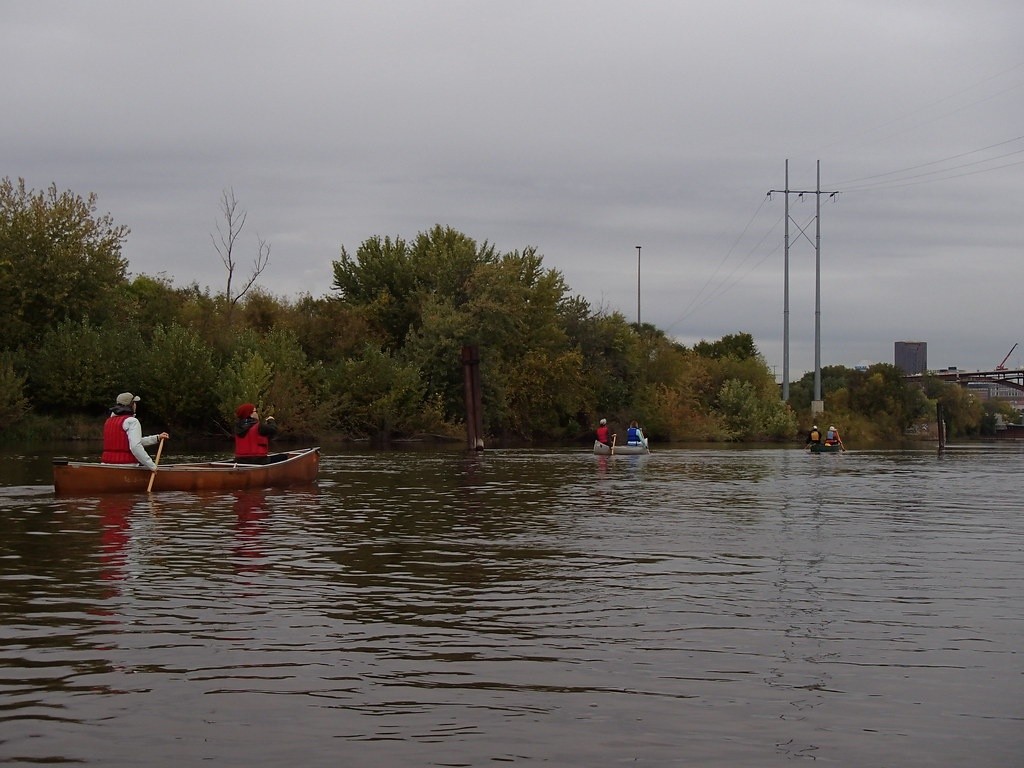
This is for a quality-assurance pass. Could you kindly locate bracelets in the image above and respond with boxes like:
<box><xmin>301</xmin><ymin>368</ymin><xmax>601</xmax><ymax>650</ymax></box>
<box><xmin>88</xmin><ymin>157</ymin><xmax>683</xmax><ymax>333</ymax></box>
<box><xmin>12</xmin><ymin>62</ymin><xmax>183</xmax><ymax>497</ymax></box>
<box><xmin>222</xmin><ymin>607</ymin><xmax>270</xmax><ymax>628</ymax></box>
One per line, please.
<box><xmin>157</xmin><ymin>434</ymin><xmax>162</xmax><ymax>442</ymax></box>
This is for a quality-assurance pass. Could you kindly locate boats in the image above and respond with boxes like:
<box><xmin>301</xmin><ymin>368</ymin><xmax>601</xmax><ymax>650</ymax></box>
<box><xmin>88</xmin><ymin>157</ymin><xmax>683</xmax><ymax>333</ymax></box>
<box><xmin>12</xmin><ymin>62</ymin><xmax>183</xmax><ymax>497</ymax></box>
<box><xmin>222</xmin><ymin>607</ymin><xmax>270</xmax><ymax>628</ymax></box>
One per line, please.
<box><xmin>51</xmin><ymin>446</ymin><xmax>322</xmax><ymax>500</ymax></box>
<box><xmin>809</xmin><ymin>443</ymin><xmax>841</xmax><ymax>454</ymax></box>
<box><xmin>593</xmin><ymin>438</ymin><xmax>648</xmax><ymax>457</ymax></box>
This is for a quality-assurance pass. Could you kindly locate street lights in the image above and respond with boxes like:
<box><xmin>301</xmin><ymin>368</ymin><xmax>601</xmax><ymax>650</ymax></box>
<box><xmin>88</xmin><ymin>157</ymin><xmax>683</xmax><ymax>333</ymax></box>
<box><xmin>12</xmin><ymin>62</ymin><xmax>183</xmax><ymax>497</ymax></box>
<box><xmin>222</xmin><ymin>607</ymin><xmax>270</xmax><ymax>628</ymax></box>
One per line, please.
<box><xmin>635</xmin><ymin>245</ymin><xmax>642</xmax><ymax>328</ymax></box>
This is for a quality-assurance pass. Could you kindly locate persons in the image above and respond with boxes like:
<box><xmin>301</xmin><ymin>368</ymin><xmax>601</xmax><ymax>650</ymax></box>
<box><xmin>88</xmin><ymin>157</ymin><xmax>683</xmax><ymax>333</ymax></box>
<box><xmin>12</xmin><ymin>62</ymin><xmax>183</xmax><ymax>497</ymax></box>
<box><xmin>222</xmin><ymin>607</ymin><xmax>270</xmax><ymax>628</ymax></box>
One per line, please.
<box><xmin>625</xmin><ymin>421</ymin><xmax>648</xmax><ymax>447</ymax></box>
<box><xmin>596</xmin><ymin>419</ymin><xmax>617</xmax><ymax>447</ymax></box>
<box><xmin>824</xmin><ymin>426</ymin><xmax>843</xmax><ymax>445</ymax></box>
<box><xmin>100</xmin><ymin>393</ymin><xmax>169</xmax><ymax>475</ymax></box>
<box><xmin>806</xmin><ymin>426</ymin><xmax>822</xmax><ymax>446</ymax></box>
<box><xmin>234</xmin><ymin>403</ymin><xmax>288</xmax><ymax>464</ymax></box>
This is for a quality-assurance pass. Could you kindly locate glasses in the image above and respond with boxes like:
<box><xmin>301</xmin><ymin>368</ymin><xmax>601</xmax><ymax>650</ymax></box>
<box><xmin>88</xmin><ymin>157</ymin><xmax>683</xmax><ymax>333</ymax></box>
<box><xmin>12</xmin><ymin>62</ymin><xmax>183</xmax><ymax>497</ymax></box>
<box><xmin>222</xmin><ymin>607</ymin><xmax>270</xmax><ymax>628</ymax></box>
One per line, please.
<box><xmin>253</xmin><ymin>410</ymin><xmax>257</xmax><ymax>413</ymax></box>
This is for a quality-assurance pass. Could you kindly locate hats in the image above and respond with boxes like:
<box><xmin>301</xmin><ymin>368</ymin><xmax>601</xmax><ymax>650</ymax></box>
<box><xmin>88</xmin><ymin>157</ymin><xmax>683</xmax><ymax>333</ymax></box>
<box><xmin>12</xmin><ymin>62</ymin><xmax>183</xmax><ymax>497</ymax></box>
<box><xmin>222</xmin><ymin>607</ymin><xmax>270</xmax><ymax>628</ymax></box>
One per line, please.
<box><xmin>813</xmin><ymin>426</ymin><xmax>818</xmax><ymax>430</ymax></box>
<box><xmin>599</xmin><ymin>418</ymin><xmax>607</xmax><ymax>425</ymax></box>
<box><xmin>236</xmin><ymin>403</ymin><xmax>254</xmax><ymax>420</ymax></box>
<box><xmin>116</xmin><ymin>392</ymin><xmax>141</xmax><ymax>407</ymax></box>
<box><xmin>829</xmin><ymin>426</ymin><xmax>835</xmax><ymax>431</ymax></box>
